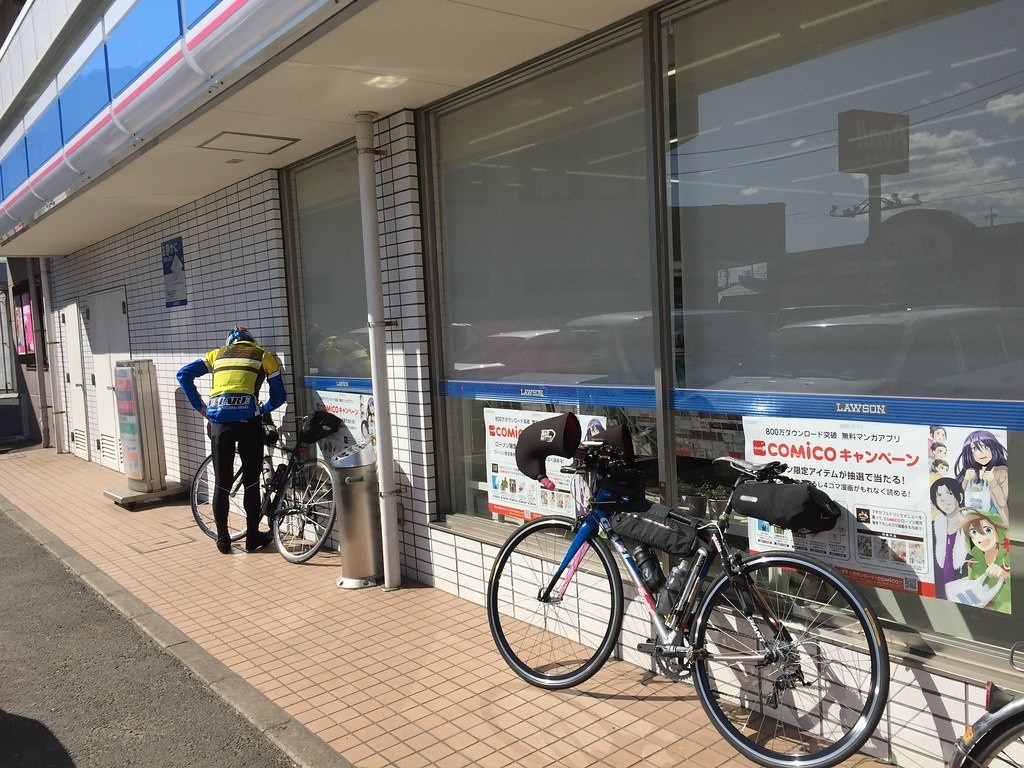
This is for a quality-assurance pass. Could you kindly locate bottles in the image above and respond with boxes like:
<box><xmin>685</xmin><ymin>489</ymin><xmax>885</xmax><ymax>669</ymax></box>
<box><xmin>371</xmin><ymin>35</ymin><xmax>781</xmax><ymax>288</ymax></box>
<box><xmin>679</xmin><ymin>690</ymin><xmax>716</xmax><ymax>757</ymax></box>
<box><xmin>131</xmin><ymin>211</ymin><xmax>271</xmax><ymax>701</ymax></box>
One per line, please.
<box><xmin>261</xmin><ymin>455</ymin><xmax>275</xmax><ymax>485</ymax></box>
<box><xmin>271</xmin><ymin>464</ymin><xmax>287</xmax><ymax>488</ymax></box>
<box><xmin>656</xmin><ymin>560</ymin><xmax>689</xmax><ymax>615</ymax></box>
<box><xmin>631</xmin><ymin>544</ymin><xmax>666</xmax><ymax>595</ymax></box>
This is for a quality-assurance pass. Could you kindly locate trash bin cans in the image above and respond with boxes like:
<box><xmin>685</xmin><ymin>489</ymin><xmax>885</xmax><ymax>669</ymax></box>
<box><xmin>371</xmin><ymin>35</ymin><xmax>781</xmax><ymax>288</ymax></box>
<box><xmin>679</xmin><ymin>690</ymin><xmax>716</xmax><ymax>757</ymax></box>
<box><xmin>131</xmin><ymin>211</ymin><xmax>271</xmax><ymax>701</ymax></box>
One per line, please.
<box><xmin>329</xmin><ymin>441</ymin><xmax>384</xmax><ymax>589</ymax></box>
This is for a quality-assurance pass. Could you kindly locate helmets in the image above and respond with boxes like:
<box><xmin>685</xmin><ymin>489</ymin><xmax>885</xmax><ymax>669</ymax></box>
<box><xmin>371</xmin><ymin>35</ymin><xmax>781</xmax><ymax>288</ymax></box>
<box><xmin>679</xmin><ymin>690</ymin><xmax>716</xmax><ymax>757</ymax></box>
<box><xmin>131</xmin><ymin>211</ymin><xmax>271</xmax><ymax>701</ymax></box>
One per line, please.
<box><xmin>225</xmin><ymin>327</ymin><xmax>257</xmax><ymax>344</ymax></box>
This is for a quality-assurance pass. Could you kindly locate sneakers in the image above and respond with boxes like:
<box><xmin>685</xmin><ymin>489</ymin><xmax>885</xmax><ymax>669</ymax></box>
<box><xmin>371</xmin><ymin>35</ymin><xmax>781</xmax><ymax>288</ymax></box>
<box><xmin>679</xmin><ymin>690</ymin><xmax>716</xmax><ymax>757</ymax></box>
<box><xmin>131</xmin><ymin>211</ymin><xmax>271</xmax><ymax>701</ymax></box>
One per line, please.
<box><xmin>217</xmin><ymin>541</ymin><xmax>231</xmax><ymax>554</ymax></box>
<box><xmin>246</xmin><ymin>530</ymin><xmax>274</xmax><ymax>551</ymax></box>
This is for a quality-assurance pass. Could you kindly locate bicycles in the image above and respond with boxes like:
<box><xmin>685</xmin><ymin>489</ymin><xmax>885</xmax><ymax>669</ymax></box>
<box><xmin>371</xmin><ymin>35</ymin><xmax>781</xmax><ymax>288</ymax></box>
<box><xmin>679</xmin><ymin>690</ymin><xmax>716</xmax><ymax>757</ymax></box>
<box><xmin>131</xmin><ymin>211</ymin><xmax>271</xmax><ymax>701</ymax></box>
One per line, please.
<box><xmin>189</xmin><ymin>414</ymin><xmax>338</xmax><ymax>564</ymax></box>
<box><xmin>484</xmin><ymin>411</ymin><xmax>892</xmax><ymax>768</ymax></box>
<box><xmin>947</xmin><ymin>641</ymin><xmax>1023</xmax><ymax>768</ymax></box>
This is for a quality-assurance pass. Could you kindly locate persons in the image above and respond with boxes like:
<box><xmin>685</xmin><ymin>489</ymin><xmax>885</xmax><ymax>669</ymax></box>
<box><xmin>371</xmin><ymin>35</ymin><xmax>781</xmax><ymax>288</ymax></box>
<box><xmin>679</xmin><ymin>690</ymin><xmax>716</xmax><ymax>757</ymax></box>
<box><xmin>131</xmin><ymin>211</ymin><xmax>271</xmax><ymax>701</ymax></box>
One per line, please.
<box><xmin>176</xmin><ymin>326</ymin><xmax>287</xmax><ymax>554</ymax></box>
<box><xmin>306</xmin><ymin>323</ymin><xmax>372</xmax><ymax>380</ymax></box>
